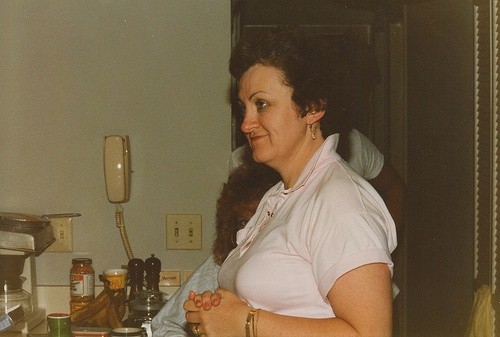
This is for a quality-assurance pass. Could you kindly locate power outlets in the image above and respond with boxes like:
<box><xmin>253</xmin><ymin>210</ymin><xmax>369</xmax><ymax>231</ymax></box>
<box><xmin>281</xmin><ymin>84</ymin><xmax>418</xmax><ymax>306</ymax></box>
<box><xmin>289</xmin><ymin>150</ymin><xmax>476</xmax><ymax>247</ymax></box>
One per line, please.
<box><xmin>45</xmin><ymin>217</ymin><xmax>73</xmax><ymax>253</ymax></box>
<box><xmin>166</xmin><ymin>214</ymin><xmax>202</xmax><ymax>250</ymax></box>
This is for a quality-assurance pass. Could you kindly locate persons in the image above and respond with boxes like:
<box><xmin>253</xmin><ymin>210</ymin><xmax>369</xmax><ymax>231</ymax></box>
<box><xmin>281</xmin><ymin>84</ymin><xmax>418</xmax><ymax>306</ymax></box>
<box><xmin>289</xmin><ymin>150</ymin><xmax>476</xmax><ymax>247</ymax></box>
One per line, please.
<box><xmin>183</xmin><ymin>23</ymin><xmax>398</xmax><ymax>337</ymax></box>
<box><xmin>212</xmin><ymin>161</ymin><xmax>283</xmax><ymax>265</ymax></box>
<box><xmin>228</xmin><ymin>86</ymin><xmax>406</xmax><ymax>280</ymax></box>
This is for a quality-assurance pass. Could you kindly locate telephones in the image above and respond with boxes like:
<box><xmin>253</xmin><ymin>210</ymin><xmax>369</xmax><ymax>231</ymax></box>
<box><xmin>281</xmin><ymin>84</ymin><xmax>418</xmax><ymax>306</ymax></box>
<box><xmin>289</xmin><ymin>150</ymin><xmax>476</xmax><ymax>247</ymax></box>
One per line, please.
<box><xmin>104</xmin><ymin>134</ymin><xmax>132</xmax><ymax>205</ymax></box>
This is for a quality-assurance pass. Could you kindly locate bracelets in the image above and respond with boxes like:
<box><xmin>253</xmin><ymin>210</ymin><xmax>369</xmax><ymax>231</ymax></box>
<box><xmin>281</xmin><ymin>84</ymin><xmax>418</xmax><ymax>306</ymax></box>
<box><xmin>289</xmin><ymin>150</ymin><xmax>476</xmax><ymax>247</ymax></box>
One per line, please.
<box><xmin>245</xmin><ymin>308</ymin><xmax>261</xmax><ymax>337</ymax></box>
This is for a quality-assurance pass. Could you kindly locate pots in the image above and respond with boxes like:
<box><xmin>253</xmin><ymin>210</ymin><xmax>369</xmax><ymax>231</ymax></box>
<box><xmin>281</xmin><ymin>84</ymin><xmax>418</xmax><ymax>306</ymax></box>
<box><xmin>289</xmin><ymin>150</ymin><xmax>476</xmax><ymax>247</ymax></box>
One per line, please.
<box><xmin>0</xmin><ymin>213</ymin><xmax>50</xmax><ymax>234</ymax></box>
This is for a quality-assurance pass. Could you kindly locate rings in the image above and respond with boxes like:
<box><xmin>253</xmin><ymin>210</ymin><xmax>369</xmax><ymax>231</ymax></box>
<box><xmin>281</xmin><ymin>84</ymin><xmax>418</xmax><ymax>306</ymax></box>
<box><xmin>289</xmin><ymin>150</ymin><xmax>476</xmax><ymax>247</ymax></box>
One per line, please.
<box><xmin>195</xmin><ymin>324</ymin><xmax>201</xmax><ymax>337</ymax></box>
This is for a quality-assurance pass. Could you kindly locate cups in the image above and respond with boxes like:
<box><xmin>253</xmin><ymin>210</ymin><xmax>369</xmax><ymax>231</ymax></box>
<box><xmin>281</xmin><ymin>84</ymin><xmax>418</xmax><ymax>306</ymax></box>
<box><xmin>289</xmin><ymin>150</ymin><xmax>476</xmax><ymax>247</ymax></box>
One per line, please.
<box><xmin>111</xmin><ymin>328</ymin><xmax>147</xmax><ymax>337</ymax></box>
<box><xmin>104</xmin><ymin>269</ymin><xmax>128</xmax><ymax>300</ymax></box>
<box><xmin>47</xmin><ymin>313</ymin><xmax>71</xmax><ymax>337</ymax></box>
